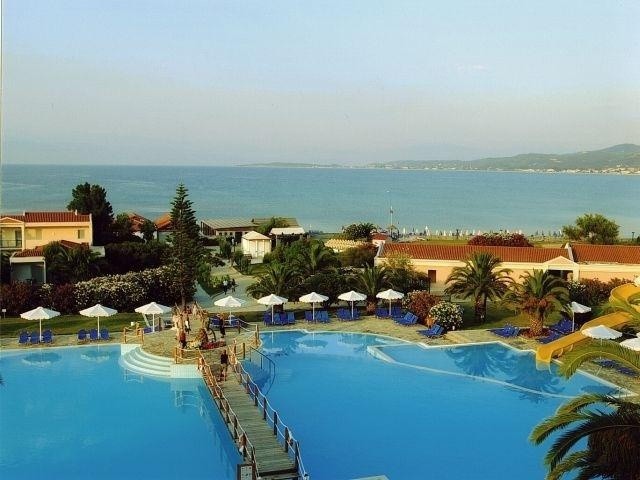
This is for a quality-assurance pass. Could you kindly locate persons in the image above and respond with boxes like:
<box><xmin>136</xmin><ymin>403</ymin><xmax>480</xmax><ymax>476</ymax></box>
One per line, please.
<box><xmin>223</xmin><ymin>279</ymin><xmax>229</xmax><ymax>295</ymax></box>
<box><xmin>179</xmin><ymin>301</ymin><xmax>198</xmax><ymax>349</ymax></box>
<box><xmin>219</xmin><ymin>314</ymin><xmax>225</xmax><ymax>338</ymax></box>
<box><xmin>231</xmin><ymin>279</ymin><xmax>235</xmax><ymax>293</ymax></box>
<box><xmin>217</xmin><ymin>350</ymin><xmax>228</xmax><ymax>381</ymax></box>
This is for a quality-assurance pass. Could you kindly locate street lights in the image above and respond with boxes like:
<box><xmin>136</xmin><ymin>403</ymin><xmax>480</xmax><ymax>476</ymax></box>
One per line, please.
<box><xmin>386</xmin><ymin>191</ymin><xmax>393</xmax><ymax>237</ymax></box>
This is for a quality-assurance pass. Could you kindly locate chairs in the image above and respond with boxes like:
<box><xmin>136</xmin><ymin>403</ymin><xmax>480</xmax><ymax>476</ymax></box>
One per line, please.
<box><xmin>534</xmin><ymin>318</ymin><xmax>582</xmax><ymax>346</ymax></box>
<box><xmin>77</xmin><ymin>327</ymin><xmax>87</xmax><ymax>345</ymax></box>
<box><xmin>42</xmin><ymin>329</ymin><xmax>52</xmax><ymax>345</ymax></box>
<box><xmin>97</xmin><ymin>327</ymin><xmax>110</xmax><ymax>344</ymax></box>
<box><xmin>86</xmin><ymin>328</ymin><xmax>99</xmax><ymax>343</ymax></box>
<box><xmin>260</xmin><ymin>304</ymin><xmax>447</xmax><ymax>340</ymax></box>
<box><xmin>17</xmin><ymin>330</ymin><xmax>29</xmax><ymax>346</ymax></box>
<box><xmin>492</xmin><ymin>323</ymin><xmax>520</xmax><ymax>340</ymax></box>
<box><xmin>137</xmin><ymin>315</ymin><xmax>250</xmax><ymax>339</ymax></box>
<box><xmin>596</xmin><ymin>356</ymin><xmax>637</xmax><ymax>377</ymax></box>
<box><xmin>30</xmin><ymin>331</ymin><xmax>41</xmax><ymax>346</ymax></box>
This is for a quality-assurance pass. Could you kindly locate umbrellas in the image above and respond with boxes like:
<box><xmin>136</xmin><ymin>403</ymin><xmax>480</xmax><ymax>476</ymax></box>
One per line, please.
<box><xmin>560</xmin><ymin>301</ymin><xmax>591</xmax><ymax>332</ymax></box>
<box><xmin>338</xmin><ymin>334</ymin><xmax>364</xmax><ymax>349</ymax></box>
<box><xmin>134</xmin><ymin>301</ymin><xmax>172</xmax><ymax>332</ymax></box>
<box><xmin>79</xmin><ymin>303</ymin><xmax>117</xmax><ymax>339</ymax></box>
<box><xmin>22</xmin><ymin>349</ymin><xmax>62</xmax><ymax>367</ymax></box>
<box><xmin>81</xmin><ymin>346</ymin><xmax>112</xmax><ymax>363</ymax></box>
<box><xmin>300</xmin><ymin>333</ymin><xmax>328</xmax><ymax>349</ymax></box>
<box><xmin>376</xmin><ymin>288</ymin><xmax>405</xmax><ymax>316</ymax></box>
<box><xmin>582</xmin><ymin>324</ymin><xmax>622</xmax><ymax>360</ymax></box>
<box><xmin>337</xmin><ymin>290</ymin><xmax>367</xmax><ymax>317</ymax></box>
<box><xmin>619</xmin><ymin>338</ymin><xmax>640</xmax><ymax>351</ymax></box>
<box><xmin>19</xmin><ymin>306</ymin><xmax>61</xmax><ymax>343</ymax></box>
<box><xmin>263</xmin><ymin>333</ymin><xmax>282</xmax><ymax>353</ymax></box>
<box><xmin>257</xmin><ymin>294</ymin><xmax>289</xmax><ymax>324</ymax></box>
<box><xmin>299</xmin><ymin>291</ymin><xmax>329</xmax><ymax>320</ymax></box>
<box><xmin>578</xmin><ymin>385</ymin><xmax>619</xmax><ymax>396</ymax></box>
<box><xmin>214</xmin><ymin>295</ymin><xmax>246</xmax><ymax>324</ymax></box>
